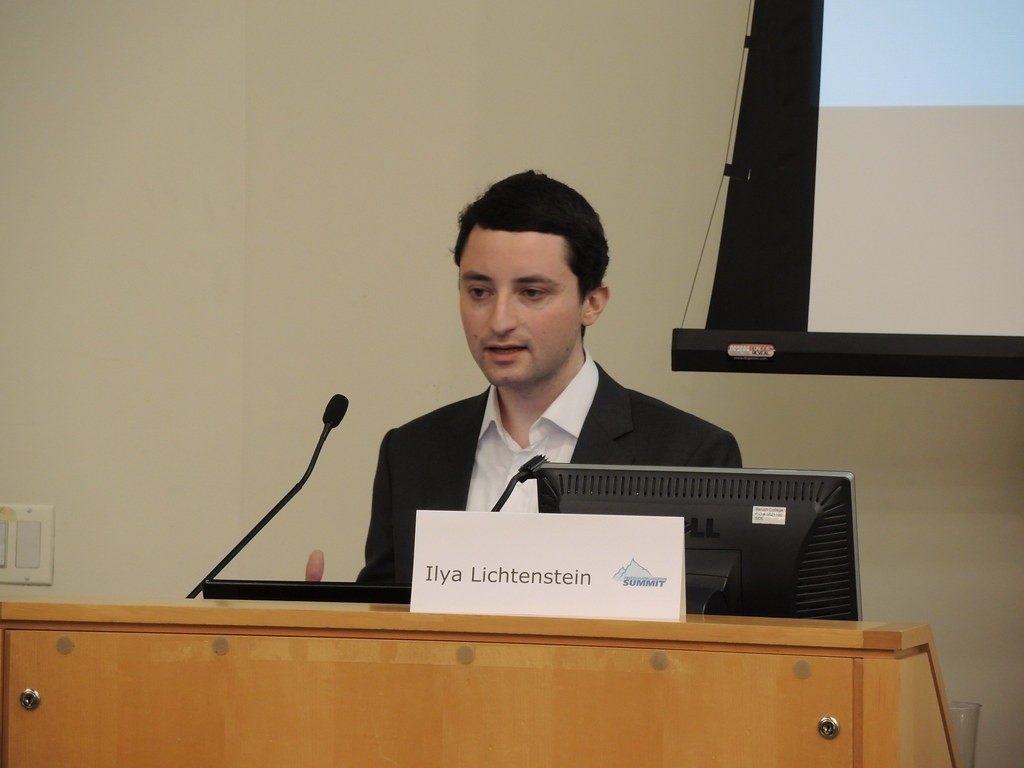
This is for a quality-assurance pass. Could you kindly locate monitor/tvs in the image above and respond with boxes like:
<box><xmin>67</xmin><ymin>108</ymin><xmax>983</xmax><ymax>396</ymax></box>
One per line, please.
<box><xmin>538</xmin><ymin>463</ymin><xmax>863</xmax><ymax>623</ymax></box>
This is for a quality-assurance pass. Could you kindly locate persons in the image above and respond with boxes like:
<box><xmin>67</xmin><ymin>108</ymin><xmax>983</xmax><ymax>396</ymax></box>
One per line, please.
<box><xmin>304</xmin><ymin>169</ymin><xmax>743</xmax><ymax>581</ymax></box>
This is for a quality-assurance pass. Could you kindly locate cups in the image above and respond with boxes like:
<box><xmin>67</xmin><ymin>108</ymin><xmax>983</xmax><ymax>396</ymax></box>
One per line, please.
<box><xmin>950</xmin><ymin>701</ymin><xmax>982</xmax><ymax>768</ymax></box>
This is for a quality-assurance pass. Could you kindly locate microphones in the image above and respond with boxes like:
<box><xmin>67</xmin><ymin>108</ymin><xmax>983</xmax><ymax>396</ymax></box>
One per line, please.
<box><xmin>491</xmin><ymin>456</ymin><xmax>551</xmax><ymax>513</ymax></box>
<box><xmin>186</xmin><ymin>393</ymin><xmax>350</xmax><ymax>599</ymax></box>
